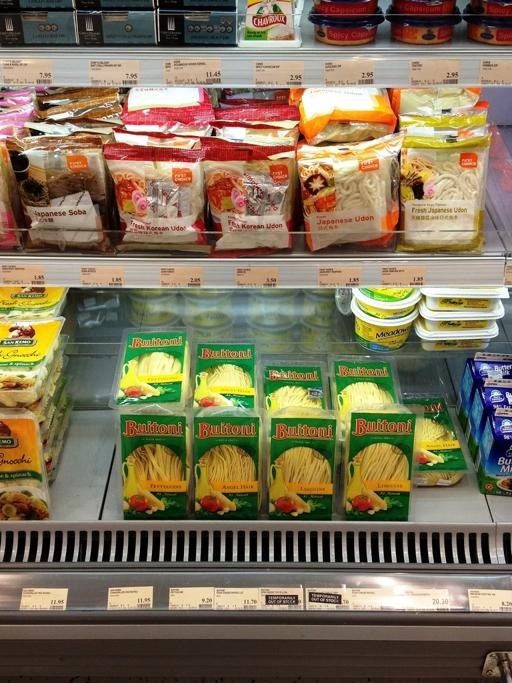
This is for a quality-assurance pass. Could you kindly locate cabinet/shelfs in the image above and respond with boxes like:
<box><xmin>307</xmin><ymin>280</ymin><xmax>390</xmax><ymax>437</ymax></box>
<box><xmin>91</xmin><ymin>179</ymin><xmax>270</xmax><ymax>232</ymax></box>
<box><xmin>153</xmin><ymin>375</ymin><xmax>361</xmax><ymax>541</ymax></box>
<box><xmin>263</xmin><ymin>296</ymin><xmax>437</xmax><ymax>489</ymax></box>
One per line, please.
<box><xmin>1</xmin><ymin>47</ymin><xmax>512</xmax><ymax>683</ymax></box>
